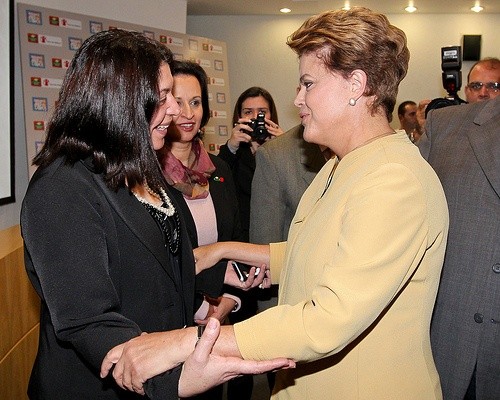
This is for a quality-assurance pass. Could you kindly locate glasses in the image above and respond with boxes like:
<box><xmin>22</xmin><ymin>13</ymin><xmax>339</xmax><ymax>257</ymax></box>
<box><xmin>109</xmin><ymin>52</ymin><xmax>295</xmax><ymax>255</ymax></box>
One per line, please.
<box><xmin>467</xmin><ymin>82</ymin><xmax>500</xmax><ymax>93</ymax></box>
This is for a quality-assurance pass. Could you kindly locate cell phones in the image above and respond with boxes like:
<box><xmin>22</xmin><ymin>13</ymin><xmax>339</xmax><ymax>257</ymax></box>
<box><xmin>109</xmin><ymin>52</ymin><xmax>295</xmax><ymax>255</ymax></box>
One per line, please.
<box><xmin>232</xmin><ymin>261</ymin><xmax>267</xmax><ymax>282</ymax></box>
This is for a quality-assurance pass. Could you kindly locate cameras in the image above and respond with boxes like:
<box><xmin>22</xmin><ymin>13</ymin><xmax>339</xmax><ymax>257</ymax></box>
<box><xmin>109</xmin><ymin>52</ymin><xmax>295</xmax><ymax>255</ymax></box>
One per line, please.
<box><xmin>424</xmin><ymin>46</ymin><xmax>470</xmax><ymax>120</ymax></box>
<box><xmin>242</xmin><ymin>113</ymin><xmax>272</xmax><ymax>141</ymax></box>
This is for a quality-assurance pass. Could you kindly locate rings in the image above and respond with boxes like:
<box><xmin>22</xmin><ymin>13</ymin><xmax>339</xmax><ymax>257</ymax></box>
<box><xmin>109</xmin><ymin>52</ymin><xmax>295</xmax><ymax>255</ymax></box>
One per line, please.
<box><xmin>249</xmin><ymin>285</ymin><xmax>254</xmax><ymax>289</ymax></box>
<box><xmin>259</xmin><ymin>284</ymin><xmax>263</xmax><ymax>288</ymax></box>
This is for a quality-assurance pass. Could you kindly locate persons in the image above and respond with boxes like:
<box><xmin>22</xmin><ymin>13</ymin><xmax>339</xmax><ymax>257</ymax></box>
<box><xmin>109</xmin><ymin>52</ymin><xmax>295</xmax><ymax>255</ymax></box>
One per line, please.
<box><xmin>19</xmin><ymin>6</ymin><xmax>500</xmax><ymax>400</ymax></box>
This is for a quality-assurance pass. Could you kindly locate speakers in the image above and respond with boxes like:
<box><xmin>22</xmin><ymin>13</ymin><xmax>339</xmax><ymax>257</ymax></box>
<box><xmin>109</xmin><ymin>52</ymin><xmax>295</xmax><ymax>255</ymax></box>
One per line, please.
<box><xmin>463</xmin><ymin>35</ymin><xmax>481</xmax><ymax>61</ymax></box>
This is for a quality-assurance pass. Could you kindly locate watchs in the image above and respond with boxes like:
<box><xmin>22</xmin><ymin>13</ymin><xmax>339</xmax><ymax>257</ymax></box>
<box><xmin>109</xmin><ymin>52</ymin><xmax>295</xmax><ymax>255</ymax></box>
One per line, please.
<box><xmin>232</xmin><ymin>301</ymin><xmax>239</xmax><ymax>310</ymax></box>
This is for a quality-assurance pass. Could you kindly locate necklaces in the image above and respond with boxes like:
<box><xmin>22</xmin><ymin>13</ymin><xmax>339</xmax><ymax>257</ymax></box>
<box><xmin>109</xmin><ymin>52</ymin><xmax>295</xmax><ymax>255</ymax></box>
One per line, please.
<box><xmin>127</xmin><ymin>178</ymin><xmax>180</xmax><ymax>256</ymax></box>
<box><xmin>326</xmin><ymin>131</ymin><xmax>397</xmax><ymax>190</ymax></box>
